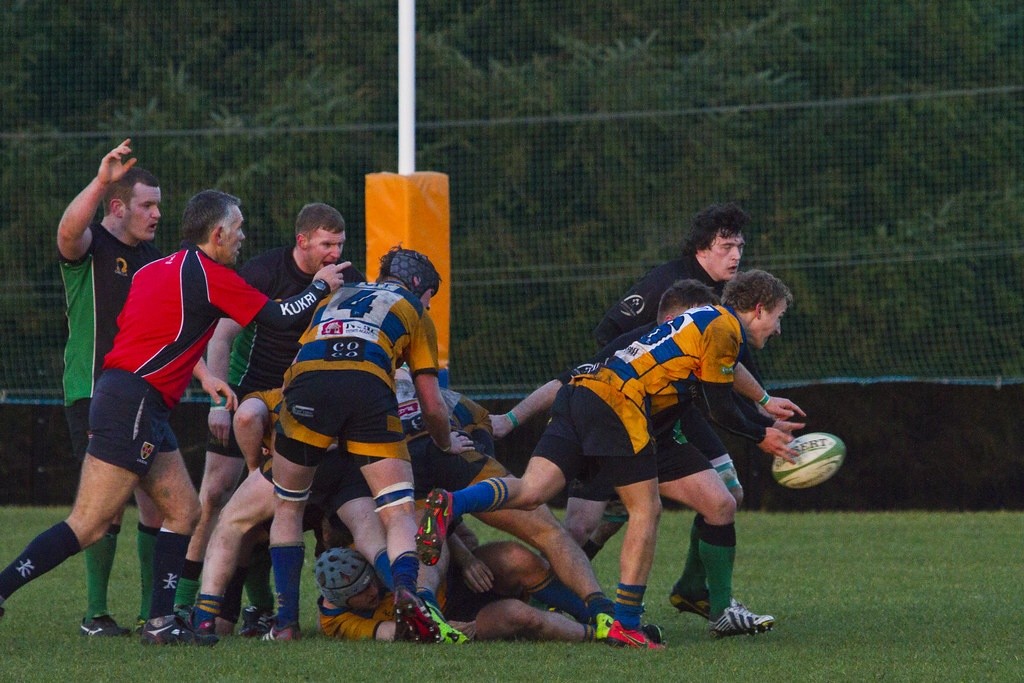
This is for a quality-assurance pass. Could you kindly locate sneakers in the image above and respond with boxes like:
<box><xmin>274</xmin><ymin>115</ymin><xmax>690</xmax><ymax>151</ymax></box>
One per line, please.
<box><xmin>708</xmin><ymin>598</ymin><xmax>775</xmax><ymax>638</ymax></box>
<box><xmin>138</xmin><ymin>618</ymin><xmax>219</xmax><ymax>647</ymax></box>
<box><xmin>414</xmin><ymin>488</ymin><xmax>463</xmax><ymax>566</ymax></box>
<box><xmin>595</xmin><ymin>613</ymin><xmax>615</xmax><ymax>641</ymax></box>
<box><xmin>238</xmin><ymin>614</ymin><xmax>275</xmax><ymax>636</ymax></box>
<box><xmin>426</xmin><ymin>602</ymin><xmax>469</xmax><ymax>644</ymax></box>
<box><xmin>173</xmin><ymin>604</ymin><xmax>220</xmax><ymax>635</ymax></box>
<box><xmin>79</xmin><ymin>615</ymin><xmax>131</xmax><ymax>638</ymax></box>
<box><xmin>604</xmin><ymin>620</ymin><xmax>665</xmax><ymax>650</ymax></box>
<box><xmin>669</xmin><ymin>580</ymin><xmax>712</xmax><ymax>621</ymax></box>
<box><xmin>392</xmin><ymin>588</ymin><xmax>441</xmax><ymax>644</ymax></box>
<box><xmin>259</xmin><ymin>622</ymin><xmax>302</xmax><ymax>642</ymax></box>
<box><xmin>641</xmin><ymin>622</ymin><xmax>662</xmax><ymax>644</ymax></box>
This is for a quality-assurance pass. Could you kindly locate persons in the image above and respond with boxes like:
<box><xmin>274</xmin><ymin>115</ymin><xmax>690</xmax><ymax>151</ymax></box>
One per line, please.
<box><xmin>0</xmin><ymin>188</ymin><xmax>352</xmax><ymax>647</ymax></box>
<box><xmin>172</xmin><ymin>202</ymin><xmax>807</xmax><ymax>647</ymax></box>
<box><xmin>54</xmin><ymin>139</ymin><xmax>165</xmax><ymax>634</ymax></box>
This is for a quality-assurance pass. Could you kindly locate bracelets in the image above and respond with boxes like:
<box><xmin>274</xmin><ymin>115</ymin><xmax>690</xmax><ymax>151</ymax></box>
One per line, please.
<box><xmin>209</xmin><ymin>396</ymin><xmax>231</xmax><ymax>411</ymax></box>
<box><xmin>507</xmin><ymin>411</ymin><xmax>519</xmax><ymax>428</ymax></box>
<box><xmin>430</xmin><ymin>436</ymin><xmax>450</xmax><ymax>453</ymax></box>
<box><xmin>759</xmin><ymin>392</ymin><xmax>770</xmax><ymax>405</ymax></box>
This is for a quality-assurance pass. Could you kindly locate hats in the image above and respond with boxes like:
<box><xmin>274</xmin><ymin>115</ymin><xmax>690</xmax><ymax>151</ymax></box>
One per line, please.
<box><xmin>313</xmin><ymin>546</ymin><xmax>380</xmax><ymax>610</ymax></box>
<box><xmin>377</xmin><ymin>248</ymin><xmax>438</xmax><ymax>298</ymax></box>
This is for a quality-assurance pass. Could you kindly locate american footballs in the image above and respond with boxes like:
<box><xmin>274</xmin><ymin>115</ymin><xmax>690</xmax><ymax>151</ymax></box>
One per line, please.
<box><xmin>770</xmin><ymin>431</ymin><xmax>849</xmax><ymax>490</ymax></box>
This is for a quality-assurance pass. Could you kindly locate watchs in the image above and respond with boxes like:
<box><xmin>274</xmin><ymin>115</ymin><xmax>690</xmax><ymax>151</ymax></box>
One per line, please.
<box><xmin>311</xmin><ymin>280</ymin><xmax>328</xmax><ymax>299</ymax></box>
